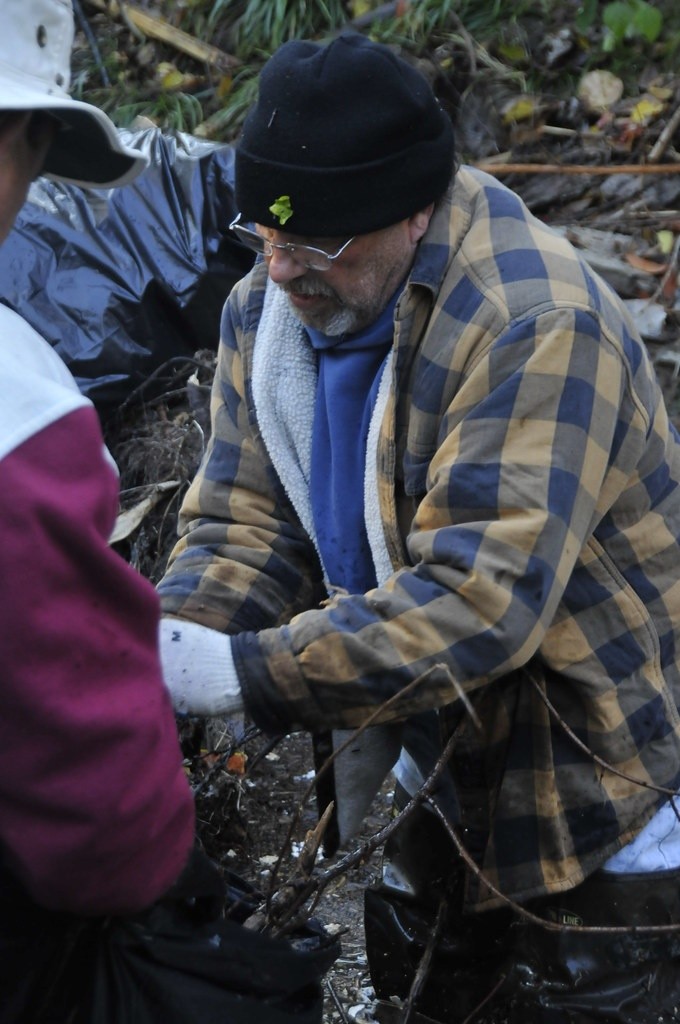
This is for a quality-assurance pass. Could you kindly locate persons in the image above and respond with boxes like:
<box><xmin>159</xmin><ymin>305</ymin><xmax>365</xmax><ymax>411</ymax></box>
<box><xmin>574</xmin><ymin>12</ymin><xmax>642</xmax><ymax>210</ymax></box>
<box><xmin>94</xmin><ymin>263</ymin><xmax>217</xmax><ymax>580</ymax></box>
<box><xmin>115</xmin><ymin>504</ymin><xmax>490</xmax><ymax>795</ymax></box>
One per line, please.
<box><xmin>1</xmin><ymin>3</ymin><xmax>196</xmax><ymax>1021</ymax></box>
<box><xmin>153</xmin><ymin>37</ymin><xmax>679</xmax><ymax>1021</ymax></box>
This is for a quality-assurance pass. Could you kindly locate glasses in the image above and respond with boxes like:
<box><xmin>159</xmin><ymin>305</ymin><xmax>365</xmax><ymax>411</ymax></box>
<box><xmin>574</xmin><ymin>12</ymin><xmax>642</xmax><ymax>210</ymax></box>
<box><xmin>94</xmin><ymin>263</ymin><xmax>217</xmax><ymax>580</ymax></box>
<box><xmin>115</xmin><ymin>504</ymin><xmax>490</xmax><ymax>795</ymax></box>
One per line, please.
<box><xmin>230</xmin><ymin>212</ymin><xmax>356</xmax><ymax>272</ymax></box>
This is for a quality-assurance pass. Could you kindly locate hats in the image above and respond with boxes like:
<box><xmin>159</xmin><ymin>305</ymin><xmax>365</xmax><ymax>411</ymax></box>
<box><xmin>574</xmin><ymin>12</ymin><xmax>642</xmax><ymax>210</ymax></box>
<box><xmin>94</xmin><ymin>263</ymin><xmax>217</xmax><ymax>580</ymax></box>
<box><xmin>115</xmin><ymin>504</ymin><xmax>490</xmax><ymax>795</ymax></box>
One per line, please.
<box><xmin>0</xmin><ymin>0</ymin><xmax>149</xmax><ymax>190</ymax></box>
<box><xmin>234</xmin><ymin>31</ymin><xmax>455</xmax><ymax>237</ymax></box>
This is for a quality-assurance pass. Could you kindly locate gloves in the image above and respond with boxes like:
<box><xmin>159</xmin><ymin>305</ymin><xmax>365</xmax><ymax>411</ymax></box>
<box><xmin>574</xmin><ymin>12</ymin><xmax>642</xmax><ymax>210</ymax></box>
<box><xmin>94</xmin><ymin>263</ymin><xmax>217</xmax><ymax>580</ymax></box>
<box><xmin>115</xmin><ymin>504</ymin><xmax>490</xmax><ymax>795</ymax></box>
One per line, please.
<box><xmin>159</xmin><ymin>617</ymin><xmax>243</xmax><ymax>718</ymax></box>
